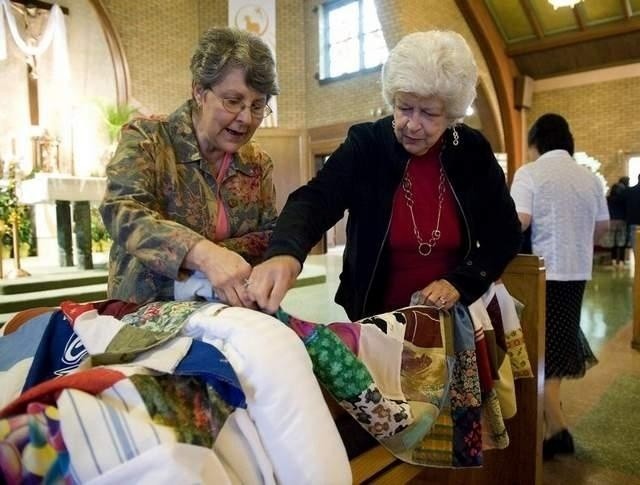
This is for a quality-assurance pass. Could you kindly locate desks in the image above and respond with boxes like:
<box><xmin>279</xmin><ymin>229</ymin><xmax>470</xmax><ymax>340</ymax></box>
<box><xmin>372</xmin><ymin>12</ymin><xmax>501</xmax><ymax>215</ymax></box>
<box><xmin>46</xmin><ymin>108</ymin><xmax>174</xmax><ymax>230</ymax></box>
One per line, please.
<box><xmin>14</xmin><ymin>176</ymin><xmax>108</xmax><ymax>270</ymax></box>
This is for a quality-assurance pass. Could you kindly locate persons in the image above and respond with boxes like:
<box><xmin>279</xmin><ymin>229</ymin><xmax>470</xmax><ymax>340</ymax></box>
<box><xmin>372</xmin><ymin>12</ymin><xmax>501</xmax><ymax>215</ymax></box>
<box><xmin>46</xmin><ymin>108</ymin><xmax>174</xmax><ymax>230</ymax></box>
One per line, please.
<box><xmin>99</xmin><ymin>27</ymin><xmax>281</xmax><ymax>310</ymax></box>
<box><xmin>247</xmin><ymin>29</ymin><xmax>524</xmax><ymax>323</ymax></box>
<box><xmin>606</xmin><ymin>175</ymin><xmax>640</xmax><ymax>255</ymax></box>
<box><xmin>508</xmin><ymin>114</ymin><xmax>612</xmax><ymax>461</ymax></box>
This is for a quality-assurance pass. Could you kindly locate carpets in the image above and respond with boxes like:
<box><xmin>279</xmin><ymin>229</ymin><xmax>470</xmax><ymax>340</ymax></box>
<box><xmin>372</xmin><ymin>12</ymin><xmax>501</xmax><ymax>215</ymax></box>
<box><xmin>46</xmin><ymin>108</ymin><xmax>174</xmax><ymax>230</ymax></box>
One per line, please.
<box><xmin>571</xmin><ymin>371</ymin><xmax>639</xmax><ymax>477</ymax></box>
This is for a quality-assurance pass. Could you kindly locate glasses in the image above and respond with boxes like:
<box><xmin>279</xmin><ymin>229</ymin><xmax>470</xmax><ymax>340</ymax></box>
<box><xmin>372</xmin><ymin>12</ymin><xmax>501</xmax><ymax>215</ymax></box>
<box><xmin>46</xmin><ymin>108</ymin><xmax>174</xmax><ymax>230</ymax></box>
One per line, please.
<box><xmin>207</xmin><ymin>85</ymin><xmax>273</xmax><ymax>119</ymax></box>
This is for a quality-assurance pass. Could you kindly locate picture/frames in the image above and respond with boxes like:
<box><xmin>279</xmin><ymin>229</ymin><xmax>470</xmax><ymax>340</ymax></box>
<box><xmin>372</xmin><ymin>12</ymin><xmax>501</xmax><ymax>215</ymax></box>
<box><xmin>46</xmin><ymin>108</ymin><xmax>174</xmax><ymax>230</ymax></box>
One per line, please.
<box><xmin>31</xmin><ymin>136</ymin><xmax>63</xmax><ymax>174</ymax></box>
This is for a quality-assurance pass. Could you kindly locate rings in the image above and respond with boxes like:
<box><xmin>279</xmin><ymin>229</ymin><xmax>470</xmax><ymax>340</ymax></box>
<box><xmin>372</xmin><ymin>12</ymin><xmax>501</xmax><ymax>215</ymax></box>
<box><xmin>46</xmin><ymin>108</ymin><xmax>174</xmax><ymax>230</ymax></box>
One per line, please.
<box><xmin>439</xmin><ymin>297</ymin><xmax>446</xmax><ymax>304</ymax></box>
<box><xmin>244</xmin><ymin>278</ymin><xmax>250</xmax><ymax>288</ymax></box>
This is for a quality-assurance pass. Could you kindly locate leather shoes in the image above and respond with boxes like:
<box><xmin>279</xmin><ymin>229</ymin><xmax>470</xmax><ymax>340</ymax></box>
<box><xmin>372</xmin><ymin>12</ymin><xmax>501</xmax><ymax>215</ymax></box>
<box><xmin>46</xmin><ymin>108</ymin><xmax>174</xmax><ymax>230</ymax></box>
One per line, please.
<box><xmin>543</xmin><ymin>429</ymin><xmax>575</xmax><ymax>461</ymax></box>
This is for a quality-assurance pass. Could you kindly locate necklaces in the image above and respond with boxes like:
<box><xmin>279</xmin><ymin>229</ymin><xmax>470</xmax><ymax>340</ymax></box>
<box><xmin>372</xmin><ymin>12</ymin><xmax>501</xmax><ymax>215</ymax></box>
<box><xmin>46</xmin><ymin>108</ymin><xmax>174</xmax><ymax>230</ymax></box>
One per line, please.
<box><xmin>402</xmin><ymin>167</ymin><xmax>447</xmax><ymax>256</ymax></box>
